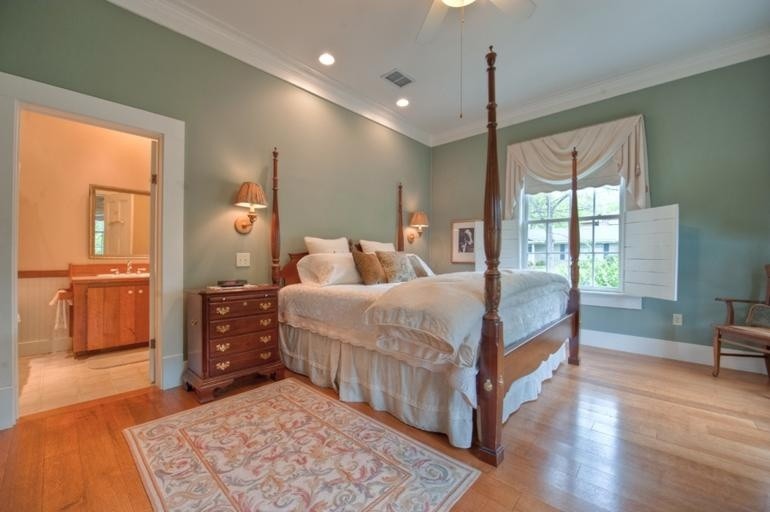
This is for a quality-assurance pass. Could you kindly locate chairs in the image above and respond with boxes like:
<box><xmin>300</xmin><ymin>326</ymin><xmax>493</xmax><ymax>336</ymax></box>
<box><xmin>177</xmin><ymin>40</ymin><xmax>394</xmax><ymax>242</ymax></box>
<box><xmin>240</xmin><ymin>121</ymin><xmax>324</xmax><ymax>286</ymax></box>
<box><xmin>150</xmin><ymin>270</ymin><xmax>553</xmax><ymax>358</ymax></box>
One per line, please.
<box><xmin>713</xmin><ymin>261</ymin><xmax>770</xmax><ymax>376</ymax></box>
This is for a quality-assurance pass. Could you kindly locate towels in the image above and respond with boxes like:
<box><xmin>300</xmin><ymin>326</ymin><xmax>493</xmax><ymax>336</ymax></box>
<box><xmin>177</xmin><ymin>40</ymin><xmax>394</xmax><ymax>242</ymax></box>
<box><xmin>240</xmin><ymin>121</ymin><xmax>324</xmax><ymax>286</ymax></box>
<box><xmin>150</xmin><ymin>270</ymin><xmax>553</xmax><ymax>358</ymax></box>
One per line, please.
<box><xmin>48</xmin><ymin>290</ymin><xmax>74</xmax><ymax>307</ymax></box>
<box><xmin>54</xmin><ymin>298</ymin><xmax>68</xmax><ymax>330</ymax></box>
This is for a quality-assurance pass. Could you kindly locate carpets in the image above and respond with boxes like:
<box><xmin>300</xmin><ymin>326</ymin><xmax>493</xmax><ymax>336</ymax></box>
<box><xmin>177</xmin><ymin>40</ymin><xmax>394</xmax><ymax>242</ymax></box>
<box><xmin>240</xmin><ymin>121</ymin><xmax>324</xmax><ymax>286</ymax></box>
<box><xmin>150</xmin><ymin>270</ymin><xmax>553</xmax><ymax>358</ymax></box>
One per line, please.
<box><xmin>82</xmin><ymin>346</ymin><xmax>152</xmax><ymax>370</ymax></box>
<box><xmin>121</xmin><ymin>378</ymin><xmax>482</xmax><ymax>512</ymax></box>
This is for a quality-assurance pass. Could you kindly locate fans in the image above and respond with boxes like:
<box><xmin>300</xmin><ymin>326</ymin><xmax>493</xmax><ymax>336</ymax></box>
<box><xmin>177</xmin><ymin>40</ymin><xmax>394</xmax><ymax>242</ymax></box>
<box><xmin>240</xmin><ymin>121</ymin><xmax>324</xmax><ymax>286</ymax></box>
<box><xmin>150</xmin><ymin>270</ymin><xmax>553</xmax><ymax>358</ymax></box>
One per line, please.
<box><xmin>418</xmin><ymin>0</ymin><xmax>537</xmax><ymax>42</ymax></box>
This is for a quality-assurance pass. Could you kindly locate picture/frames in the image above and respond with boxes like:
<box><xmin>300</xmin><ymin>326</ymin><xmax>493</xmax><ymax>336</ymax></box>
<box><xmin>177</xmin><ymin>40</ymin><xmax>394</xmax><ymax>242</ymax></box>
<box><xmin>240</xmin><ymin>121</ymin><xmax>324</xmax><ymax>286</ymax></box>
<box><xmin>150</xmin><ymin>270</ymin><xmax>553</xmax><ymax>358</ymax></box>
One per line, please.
<box><xmin>450</xmin><ymin>220</ymin><xmax>478</xmax><ymax>265</ymax></box>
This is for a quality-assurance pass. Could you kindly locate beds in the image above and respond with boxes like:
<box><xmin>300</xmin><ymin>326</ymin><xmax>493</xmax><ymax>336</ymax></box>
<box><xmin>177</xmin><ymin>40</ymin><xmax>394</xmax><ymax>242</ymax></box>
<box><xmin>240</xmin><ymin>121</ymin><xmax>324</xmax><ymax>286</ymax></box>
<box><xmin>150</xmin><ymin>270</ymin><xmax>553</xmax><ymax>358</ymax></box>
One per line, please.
<box><xmin>270</xmin><ymin>46</ymin><xmax>580</xmax><ymax>469</ymax></box>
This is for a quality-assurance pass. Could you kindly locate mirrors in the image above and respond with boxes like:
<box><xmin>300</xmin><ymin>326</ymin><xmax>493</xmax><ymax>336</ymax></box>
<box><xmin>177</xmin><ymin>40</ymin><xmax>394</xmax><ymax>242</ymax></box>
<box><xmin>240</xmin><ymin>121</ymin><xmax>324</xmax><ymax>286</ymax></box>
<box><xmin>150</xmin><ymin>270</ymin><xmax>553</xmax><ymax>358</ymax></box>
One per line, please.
<box><xmin>90</xmin><ymin>183</ymin><xmax>154</xmax><ymax>257</ymax></box>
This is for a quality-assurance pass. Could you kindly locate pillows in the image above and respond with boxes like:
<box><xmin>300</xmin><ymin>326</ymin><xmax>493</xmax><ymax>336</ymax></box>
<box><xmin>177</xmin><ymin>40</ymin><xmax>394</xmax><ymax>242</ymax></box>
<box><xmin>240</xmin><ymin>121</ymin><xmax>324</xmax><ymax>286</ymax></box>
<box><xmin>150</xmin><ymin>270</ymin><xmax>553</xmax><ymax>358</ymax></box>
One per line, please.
<box><xmin>296</xmin><ymin>234</ymin><xmax>437</xmax><ymax>291</ymax></box>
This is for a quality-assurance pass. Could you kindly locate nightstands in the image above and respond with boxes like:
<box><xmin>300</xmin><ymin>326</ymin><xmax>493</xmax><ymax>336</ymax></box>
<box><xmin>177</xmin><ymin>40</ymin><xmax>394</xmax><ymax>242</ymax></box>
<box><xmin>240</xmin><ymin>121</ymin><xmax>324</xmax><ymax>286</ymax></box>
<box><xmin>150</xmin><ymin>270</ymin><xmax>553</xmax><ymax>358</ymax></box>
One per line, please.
<box><xmin>183</xmin><ymin>283</ymin><xmax>285</xmax><ymax>404</ymax></box>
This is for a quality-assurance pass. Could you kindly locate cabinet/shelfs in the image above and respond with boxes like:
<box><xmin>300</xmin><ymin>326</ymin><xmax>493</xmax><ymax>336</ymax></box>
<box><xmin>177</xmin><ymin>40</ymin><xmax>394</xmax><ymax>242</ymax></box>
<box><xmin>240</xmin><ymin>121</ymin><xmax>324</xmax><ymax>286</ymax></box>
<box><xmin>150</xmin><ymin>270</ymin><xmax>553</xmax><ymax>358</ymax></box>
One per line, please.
<box><xmin>70</xmin><ymin>280</ymin><xmax>150</xmax><ymax>354</ymax></box>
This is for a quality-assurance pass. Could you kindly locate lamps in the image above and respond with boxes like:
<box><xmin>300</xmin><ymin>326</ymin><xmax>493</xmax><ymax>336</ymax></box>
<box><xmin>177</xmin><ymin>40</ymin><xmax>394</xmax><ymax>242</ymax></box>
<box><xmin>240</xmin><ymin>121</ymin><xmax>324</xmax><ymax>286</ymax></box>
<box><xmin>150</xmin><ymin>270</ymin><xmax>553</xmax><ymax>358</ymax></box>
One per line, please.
<box><xmin>230</xmin><ymin>180</ymin><xmax>269</xmax><ymax>236</ymax></box>
<box><xmin>405</xmin><ymin>210</ymin><xmax>433</xmax><ymax>246</ymax></box>
